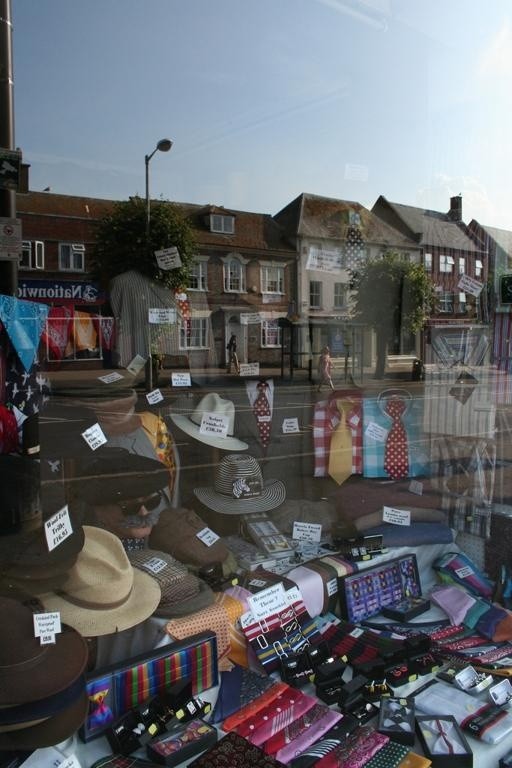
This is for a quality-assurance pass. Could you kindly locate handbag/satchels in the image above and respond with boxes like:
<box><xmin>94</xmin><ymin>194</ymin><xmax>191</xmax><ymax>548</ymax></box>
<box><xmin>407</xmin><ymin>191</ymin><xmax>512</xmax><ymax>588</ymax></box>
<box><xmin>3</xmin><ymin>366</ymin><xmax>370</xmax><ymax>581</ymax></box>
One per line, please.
<box><xmin>227</xmin><ymin>344</ymin><xmax>236</xmax><ymax>352</ymax></box>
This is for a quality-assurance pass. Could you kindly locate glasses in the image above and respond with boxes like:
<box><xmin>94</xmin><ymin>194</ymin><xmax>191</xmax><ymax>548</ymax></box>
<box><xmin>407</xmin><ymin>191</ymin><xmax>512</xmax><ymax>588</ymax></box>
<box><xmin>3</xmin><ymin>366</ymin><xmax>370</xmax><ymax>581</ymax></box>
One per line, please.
<box><xmin>115</xmin><ymin>491</ymin><xmax>160</xmax><ymax>516</ymax></box>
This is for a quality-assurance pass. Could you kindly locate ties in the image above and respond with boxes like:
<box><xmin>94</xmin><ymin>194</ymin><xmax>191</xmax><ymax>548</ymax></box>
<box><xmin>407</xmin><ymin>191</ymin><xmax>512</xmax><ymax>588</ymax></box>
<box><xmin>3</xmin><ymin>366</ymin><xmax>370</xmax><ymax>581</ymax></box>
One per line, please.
<box><xmin>175</xmin><ymin>287</ymin><xmax>193</xmax><ymax>337</ymax></box>
<box><xmin>384</xmin><ymin>396</ymin><xmax>411</xmax><ymax>478</ymax></box>
<box><xmin>252</xmin><ymin>380</ymin><xmax>276</xmax><ymax>447</ymax></box>
<box><xmin>328</xmin><ymin>399</ymin><xmax>357</xmax><ymax>486</ymax></box>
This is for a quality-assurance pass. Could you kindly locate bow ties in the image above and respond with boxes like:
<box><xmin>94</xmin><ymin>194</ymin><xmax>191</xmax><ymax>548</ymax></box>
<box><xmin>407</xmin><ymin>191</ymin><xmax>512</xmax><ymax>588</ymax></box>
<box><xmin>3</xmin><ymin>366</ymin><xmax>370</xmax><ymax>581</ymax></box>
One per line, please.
<box><xmin>386</xmin><ymin>692</ymin><xmax>415</xmax><ymax>736</ymax></box>
<box><xmin>155</xmin><ymin>719</ymin><xmax>211</xmax><ymax>756</ymax></box>
<box><xmin>400</xmin><ymin>559</ymin><xmax>421</xmax><ymax>596</ymax></box>
<box><xmin>88</xmin><ymin>682</ymin><xmax>116</xmax><ymax>734</ymax></box>
<box><xmin>423</xmin><ymin>716</ymin><xmax>466</xmax><ymax>758</ymax></box>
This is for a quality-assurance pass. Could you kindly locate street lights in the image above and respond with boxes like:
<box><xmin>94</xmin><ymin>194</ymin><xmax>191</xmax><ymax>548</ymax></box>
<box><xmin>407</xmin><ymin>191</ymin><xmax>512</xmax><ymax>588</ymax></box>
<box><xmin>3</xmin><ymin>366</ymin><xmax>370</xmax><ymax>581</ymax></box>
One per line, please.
<box><xmin>145</xmin><ymin>138</ymin><xmax>174</xmax><ymax>391</ymax></box>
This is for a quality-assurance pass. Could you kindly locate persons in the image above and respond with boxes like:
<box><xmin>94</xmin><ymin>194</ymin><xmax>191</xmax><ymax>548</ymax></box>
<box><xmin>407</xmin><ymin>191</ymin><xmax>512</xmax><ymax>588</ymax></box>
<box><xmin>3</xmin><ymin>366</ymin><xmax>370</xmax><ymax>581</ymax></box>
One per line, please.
<box><xmin>109</xmin><ymin>245</ymin><xmax>219</xmax><ymax>388</ymax></box>
<box><xmin>222</xmin><ymin>333</ymin><xmax>240</xmax><ymax>372</ymax></box>
<box><xmin>72</xmin><ymin>451</ymin><xmax>173</xmax><ymax>540</ymax></box>
<box><xmin>317</xmin><ymin>345</ymin><xmax>335</xmax><ymax>392</ymax></box>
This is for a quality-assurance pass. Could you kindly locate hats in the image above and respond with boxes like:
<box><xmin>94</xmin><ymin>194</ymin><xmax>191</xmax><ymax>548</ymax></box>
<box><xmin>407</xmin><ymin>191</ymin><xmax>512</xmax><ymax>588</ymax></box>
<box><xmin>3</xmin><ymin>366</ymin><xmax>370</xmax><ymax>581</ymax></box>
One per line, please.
<box><xmin>147</xmin><ymin>509</ymin><xmax>238</xmax><ymax>579</ymax></box>
<box><xmin>127</xmin><ymin>549</ymin><xmax>215</xmax><ymax>616</ymax></box>
<box><xmin>73</xmin><ymin>366</ymin><xmax>137</xmax><ymax>408</ymax></box>
<box><xmin>37</xmin><ymin>523</ymin><xmax>159</xmax><ymax>638</ymax></box>
<box><xmin>170</xmin><ymin>392</ymin><xmax>249</xmax><ymax>452</ymax></box>
<box><xmin>23</xmin><ymin>407</ymin><xmax>128</xmax><ymax>458</ymax></box>
<box><xmin>70</xmin><ymin>447</ymin><xmax>170</xmax><ymax>505</ymax></box>
<box><xmin>1</xmin><ymin>450</ymin><xmax>85</xmax><ymax>752</ymax></box>
<box><xmin>191</xmin><ymin>454</ymin><xmax>285</xmax><ymax>516</ymax></box>
